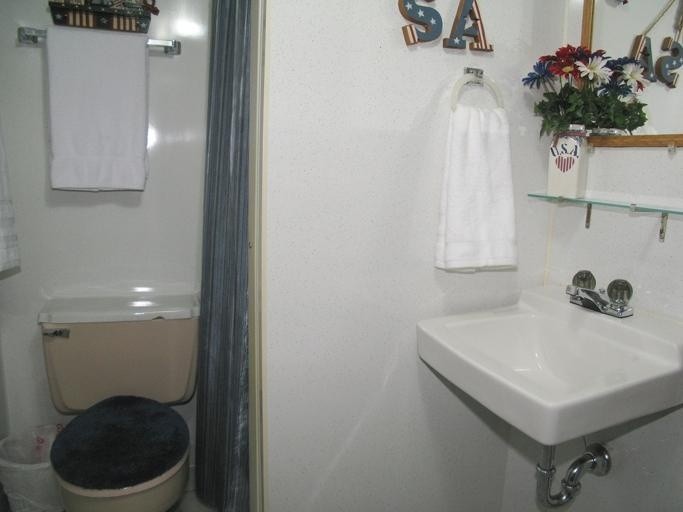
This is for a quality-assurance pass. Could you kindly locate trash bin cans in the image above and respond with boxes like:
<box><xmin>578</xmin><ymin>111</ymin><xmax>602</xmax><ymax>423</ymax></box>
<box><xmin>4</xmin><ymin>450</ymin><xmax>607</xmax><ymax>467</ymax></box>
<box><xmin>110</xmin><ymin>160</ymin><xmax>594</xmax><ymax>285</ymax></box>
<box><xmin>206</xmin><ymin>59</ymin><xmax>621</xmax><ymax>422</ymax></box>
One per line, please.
<box><xmin>0</xmin><ymin>424</ymin><xmax>65</xmax><ymax>512</ymax></box>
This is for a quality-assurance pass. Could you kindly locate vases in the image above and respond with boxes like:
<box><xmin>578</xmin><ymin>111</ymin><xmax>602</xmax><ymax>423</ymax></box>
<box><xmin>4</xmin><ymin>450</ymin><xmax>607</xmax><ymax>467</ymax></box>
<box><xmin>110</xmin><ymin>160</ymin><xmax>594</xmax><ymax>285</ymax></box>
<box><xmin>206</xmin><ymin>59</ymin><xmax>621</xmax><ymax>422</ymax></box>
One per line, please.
<box><xmin>545</xmin><ymin>123</ymin><xmax>590</xmax><ymax>197</ymax></box>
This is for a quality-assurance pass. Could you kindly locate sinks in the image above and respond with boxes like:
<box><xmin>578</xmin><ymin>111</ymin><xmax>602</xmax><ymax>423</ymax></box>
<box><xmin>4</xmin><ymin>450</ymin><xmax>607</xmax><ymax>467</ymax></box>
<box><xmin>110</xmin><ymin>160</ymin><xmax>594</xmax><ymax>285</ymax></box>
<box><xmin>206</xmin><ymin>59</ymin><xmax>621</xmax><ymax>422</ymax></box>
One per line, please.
<box><xmin>417</xmin><ymin>284</ymin><xmax>683</xmax><ymax>447</ymax></box>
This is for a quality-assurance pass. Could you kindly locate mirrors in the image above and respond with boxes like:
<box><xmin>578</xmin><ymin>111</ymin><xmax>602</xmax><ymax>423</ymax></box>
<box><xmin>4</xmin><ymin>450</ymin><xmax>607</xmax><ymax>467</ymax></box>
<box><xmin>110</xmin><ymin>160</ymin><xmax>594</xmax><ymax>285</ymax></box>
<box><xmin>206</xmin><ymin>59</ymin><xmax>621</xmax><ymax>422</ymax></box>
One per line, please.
<box><xmin>580</xmin><ymin>0</ymin><xmax>683</xmax><ymax>148</ymax></box>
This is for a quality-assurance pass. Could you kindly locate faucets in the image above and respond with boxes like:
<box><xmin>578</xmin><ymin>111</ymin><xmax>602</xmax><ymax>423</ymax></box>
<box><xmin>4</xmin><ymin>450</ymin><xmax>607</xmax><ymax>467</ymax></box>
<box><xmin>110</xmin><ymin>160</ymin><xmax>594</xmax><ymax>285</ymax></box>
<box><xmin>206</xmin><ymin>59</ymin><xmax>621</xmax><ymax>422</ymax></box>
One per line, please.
<box><xmin>565</xmin><ymin>285</ymin><xmax>607</xmax><ymax>312</ymax></box>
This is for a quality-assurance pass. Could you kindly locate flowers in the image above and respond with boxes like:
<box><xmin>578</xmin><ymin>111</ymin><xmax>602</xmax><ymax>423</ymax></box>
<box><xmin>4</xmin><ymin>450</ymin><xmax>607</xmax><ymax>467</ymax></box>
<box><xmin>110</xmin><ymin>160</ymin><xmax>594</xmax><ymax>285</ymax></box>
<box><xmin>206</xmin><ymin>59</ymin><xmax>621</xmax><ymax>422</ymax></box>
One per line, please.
<box><xmin>603</xmin><ymin>58</ymin><xmax>648</xmax><ymax>131</ymax></box>
<box><xmin>523</xmin><ymin>43</ymin><xmax>618</xmax><ymax>133</ymax></box>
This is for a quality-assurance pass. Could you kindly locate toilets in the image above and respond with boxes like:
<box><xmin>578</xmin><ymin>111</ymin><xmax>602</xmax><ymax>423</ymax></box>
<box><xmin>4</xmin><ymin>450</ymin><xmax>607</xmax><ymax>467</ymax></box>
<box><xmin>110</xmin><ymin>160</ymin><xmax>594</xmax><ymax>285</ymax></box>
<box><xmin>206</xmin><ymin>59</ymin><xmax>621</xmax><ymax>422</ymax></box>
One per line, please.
<box><xmin>36</xmin><ymin>294</ymin><xmax>203</xmax><ymax>512</ymax></box>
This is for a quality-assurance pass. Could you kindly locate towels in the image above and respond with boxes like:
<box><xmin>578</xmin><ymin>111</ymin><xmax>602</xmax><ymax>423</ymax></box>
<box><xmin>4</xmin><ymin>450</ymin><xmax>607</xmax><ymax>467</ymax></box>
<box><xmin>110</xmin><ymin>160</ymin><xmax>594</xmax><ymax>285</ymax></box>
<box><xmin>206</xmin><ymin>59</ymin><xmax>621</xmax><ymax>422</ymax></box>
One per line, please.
<box><xmin>433</xmin><ymin>102</ymin><xmax>518</xmax><ymax>275</ymax></box>
<box><xmin>44</xmin><ymin>25</ymin><xmax>149</xmax><ymax>193</ymax></box>
